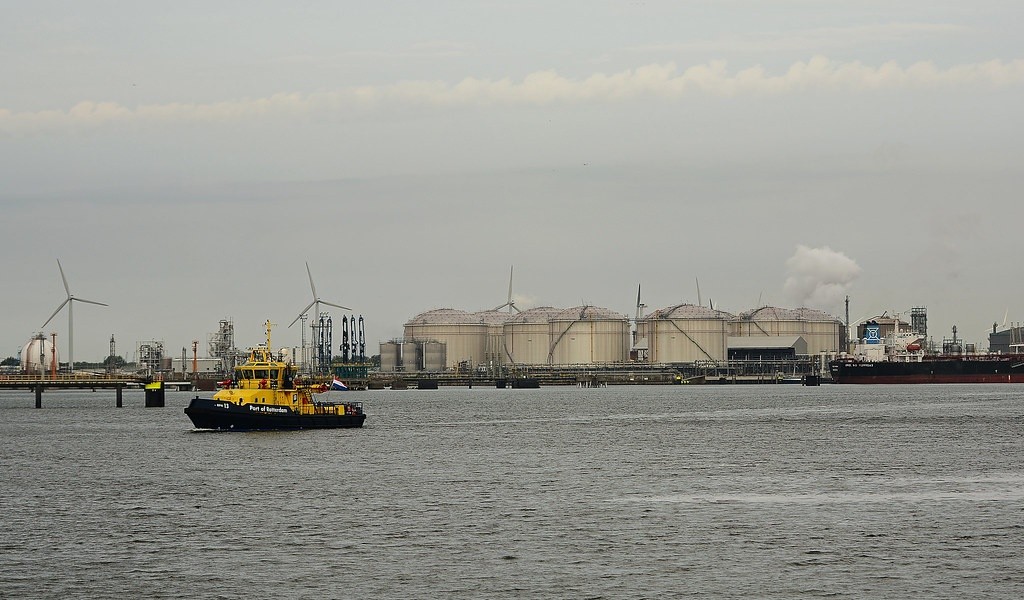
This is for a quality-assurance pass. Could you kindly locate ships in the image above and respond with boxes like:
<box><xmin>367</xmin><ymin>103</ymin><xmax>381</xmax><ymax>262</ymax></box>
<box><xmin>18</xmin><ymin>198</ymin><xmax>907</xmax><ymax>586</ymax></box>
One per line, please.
<box><xmin>828</xmin><ymin>353</ymin><xmax>1024</xmax><ymax>384</ymax></box>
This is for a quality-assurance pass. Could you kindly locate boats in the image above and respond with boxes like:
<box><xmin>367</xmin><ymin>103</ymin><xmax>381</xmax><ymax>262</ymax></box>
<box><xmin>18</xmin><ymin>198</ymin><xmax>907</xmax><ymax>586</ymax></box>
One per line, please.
<box><xmin>183</xmin><ymin>318</ymin><xmax>366</xmax><ymax>432</ymax></box>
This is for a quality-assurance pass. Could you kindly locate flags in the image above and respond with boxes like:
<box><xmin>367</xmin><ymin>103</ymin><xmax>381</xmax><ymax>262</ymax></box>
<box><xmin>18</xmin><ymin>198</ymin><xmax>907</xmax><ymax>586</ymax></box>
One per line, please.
<box><xmin>332</xmin><ymin>376</ymin><xmax>347</xmax><ymax>390</ymax></box>
